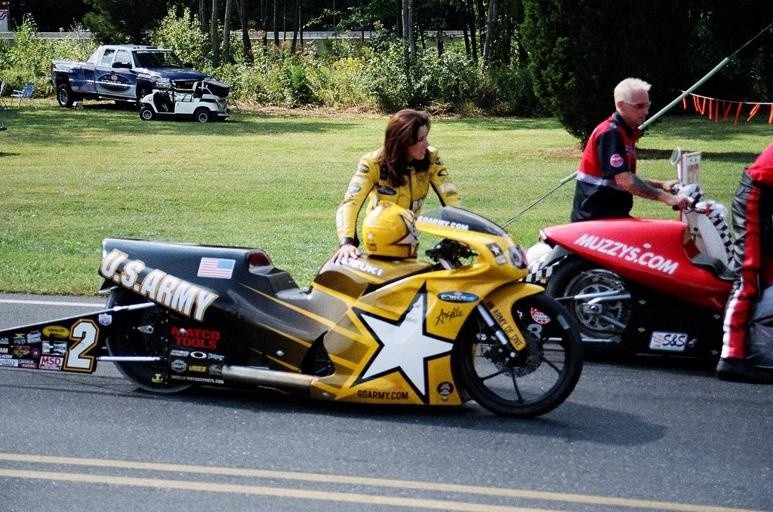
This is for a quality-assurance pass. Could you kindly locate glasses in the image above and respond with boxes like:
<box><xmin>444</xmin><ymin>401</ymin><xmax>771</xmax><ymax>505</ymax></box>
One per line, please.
<box><xmin>624</xmin><ymin>102</ymin><xmax>651</xmax><ymax>108</ymax></box>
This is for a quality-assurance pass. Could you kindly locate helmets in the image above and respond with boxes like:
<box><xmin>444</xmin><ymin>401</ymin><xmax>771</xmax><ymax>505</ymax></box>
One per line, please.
<box><xmin>361</xmin><ymin>200</ymin><xmax>421</xmax><ymax>259</ymax></box>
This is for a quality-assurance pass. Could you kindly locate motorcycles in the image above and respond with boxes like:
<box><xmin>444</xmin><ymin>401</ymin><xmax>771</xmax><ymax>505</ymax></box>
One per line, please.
<box><xmin>518</xmin><ymin>147</ymin><xmax>773</xmax><ymax>368</ymax></box>
<box><xmin>97</xmin><ymin>206</ymin><xmax>584</xmax><ymax>420</ymax></box>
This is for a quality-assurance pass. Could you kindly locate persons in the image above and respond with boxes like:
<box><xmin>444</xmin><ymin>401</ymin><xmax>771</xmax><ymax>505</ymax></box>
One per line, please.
<box><xmin>710</xmin><ymin>136</ymin><xmax>772</xmax><ymax>386</ymax></box>
<box><xmin>331</xmin><ymin>110</ymin><xmax>463</xmax><ymax>267</ymax></box>
<box><xmin>567</xmin><ymin>73</ymin><xmax>691</xmax><ymax>225</ymax></box>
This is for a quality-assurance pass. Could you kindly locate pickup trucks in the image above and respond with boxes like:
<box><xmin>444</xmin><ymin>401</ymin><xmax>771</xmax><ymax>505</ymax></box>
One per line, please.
<box><xmin>50</xmin><ymin>44</ymin><xmax>208</xmax><ymax>107</ymax></box>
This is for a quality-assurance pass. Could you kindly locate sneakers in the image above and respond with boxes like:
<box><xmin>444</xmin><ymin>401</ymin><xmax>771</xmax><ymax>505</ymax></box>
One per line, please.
<box><xmin>716</xmin><ymin>359</ymin><xmax>772</xmax><ymax>383</ymax></box>
